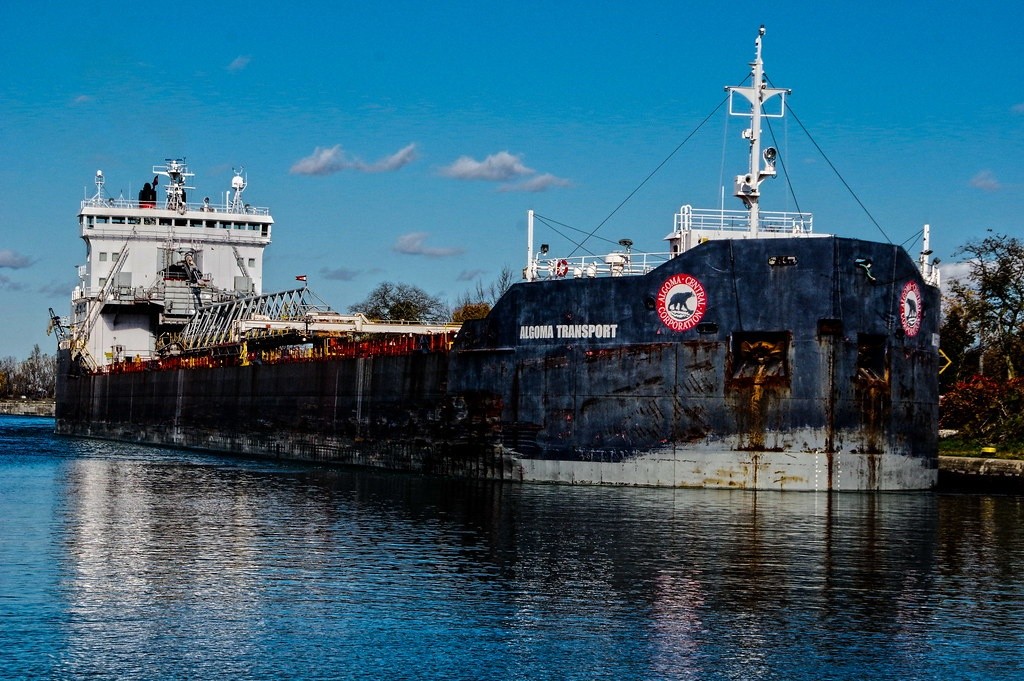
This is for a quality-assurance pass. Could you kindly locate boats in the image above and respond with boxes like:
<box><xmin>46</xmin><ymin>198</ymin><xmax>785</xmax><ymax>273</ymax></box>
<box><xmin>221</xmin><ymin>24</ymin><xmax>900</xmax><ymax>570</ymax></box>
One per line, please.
<box><xmin>47</xmin><ymin>25</ymin><xmax>941</xmax><ymax>493</ymax></box>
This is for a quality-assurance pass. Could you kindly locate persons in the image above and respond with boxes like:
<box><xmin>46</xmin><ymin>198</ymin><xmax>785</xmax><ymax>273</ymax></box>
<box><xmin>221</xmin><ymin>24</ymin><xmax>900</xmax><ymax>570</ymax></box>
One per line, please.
<box><xmin>135</xmin><ymin>354</ymin><xmax>140</xmax><ymax>371</ymax></box>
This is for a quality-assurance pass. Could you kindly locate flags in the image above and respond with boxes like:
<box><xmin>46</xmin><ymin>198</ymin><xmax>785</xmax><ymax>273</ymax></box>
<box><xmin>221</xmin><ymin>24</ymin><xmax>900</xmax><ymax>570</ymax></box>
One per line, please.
<box><xmin>152</xmin><ymin>175</ymin><xmax>158</xmax><ymax>187</ymax></box>
<box><xmin>295</xmin><ymin>275</ymin><xmax>306</xmax><ymax>281</ymax></box>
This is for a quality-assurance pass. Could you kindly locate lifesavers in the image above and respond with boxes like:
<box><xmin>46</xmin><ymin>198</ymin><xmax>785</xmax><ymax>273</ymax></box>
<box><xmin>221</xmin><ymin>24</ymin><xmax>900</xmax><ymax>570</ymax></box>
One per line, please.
<box><xmin>557</xmin><ymin>259</ymin><xmax>569</xmax><ymax>278</ymax></box>
